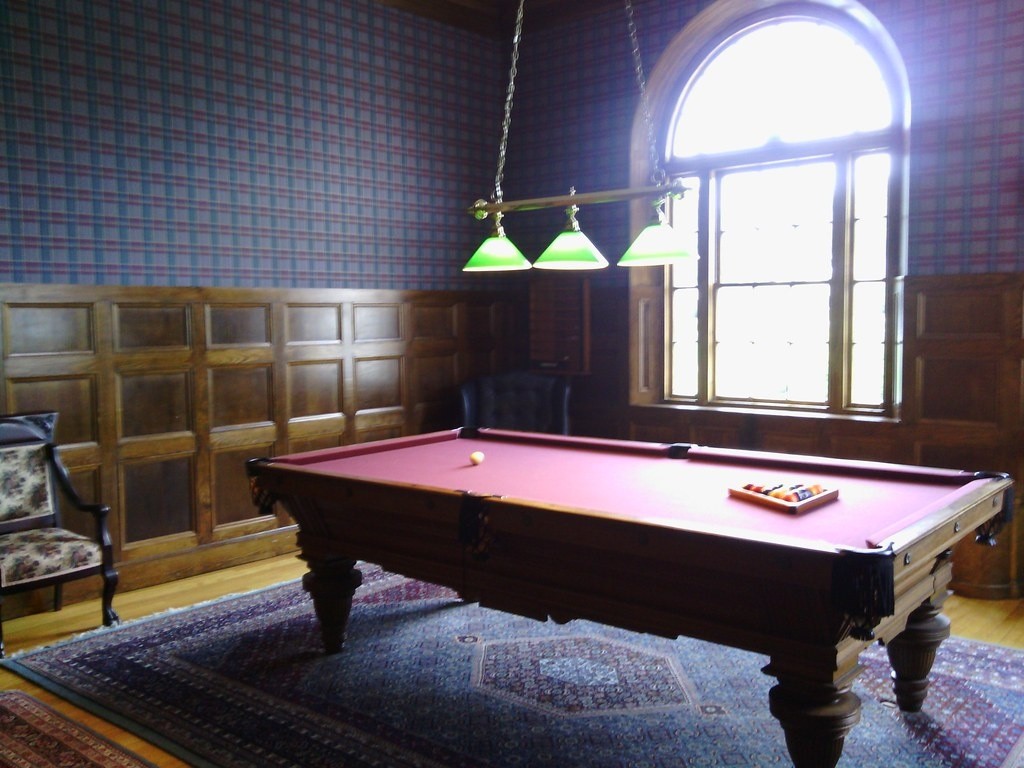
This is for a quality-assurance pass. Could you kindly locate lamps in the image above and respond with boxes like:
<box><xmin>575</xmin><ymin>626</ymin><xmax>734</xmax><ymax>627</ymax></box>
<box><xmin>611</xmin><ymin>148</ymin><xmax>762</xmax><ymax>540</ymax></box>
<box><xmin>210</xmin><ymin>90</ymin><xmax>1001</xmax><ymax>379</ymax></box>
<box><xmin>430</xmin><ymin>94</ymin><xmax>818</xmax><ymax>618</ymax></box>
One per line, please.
<box><xmin>462</xmin><ymin>0</ymin><xmax>700</xmax><ymax>271</ymax></box>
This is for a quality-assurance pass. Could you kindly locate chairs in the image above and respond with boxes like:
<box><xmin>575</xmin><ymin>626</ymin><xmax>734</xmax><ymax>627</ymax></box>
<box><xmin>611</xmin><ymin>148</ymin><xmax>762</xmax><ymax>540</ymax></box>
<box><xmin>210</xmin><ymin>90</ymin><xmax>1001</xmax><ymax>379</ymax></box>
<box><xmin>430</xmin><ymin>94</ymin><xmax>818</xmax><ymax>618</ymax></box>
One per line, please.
<box><xmin>0</xmin><ymin>411</ymin><xmax>120</xmax><ymax>658</ymax></box>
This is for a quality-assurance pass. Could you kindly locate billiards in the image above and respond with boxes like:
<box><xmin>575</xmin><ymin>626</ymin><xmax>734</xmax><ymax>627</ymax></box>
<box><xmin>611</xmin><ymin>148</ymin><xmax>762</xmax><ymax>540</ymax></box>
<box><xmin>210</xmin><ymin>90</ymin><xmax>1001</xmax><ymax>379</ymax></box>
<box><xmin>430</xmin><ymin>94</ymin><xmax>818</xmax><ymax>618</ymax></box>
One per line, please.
<box><xmin>469</xmin><ymin>452</ymin><xmax>485</xmax><ymax>465</ymax></box>
<box><xmin>743</xmin><ymin>482</ymin><xmax>823</xmax><ymax>502</ymax></box>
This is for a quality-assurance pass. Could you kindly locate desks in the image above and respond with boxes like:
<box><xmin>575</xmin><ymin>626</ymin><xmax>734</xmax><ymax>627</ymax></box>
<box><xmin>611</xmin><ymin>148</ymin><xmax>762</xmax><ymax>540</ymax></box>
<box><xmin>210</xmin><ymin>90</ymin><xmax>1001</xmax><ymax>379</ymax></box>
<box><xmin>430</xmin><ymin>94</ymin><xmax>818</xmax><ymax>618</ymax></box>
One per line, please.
<box><xmin>244</xmin><ymin>426</ymin><xmax>1015</xmax><ymax>768</ymax></box>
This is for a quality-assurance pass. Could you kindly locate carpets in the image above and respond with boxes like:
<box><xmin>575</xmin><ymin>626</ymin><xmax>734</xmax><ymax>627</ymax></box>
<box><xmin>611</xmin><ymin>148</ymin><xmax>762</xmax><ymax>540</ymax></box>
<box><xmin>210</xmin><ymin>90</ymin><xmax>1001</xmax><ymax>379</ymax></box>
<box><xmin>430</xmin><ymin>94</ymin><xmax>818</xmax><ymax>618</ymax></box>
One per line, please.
<box><xmin>1</xmin><ymin>561</ymin><xmax>1023</xmax><ymax>768</ymax></box>
<box><xmin>0</xmin><ymin>690</ymin><xmax>159</xmax><ymax>768</ymax></box>
